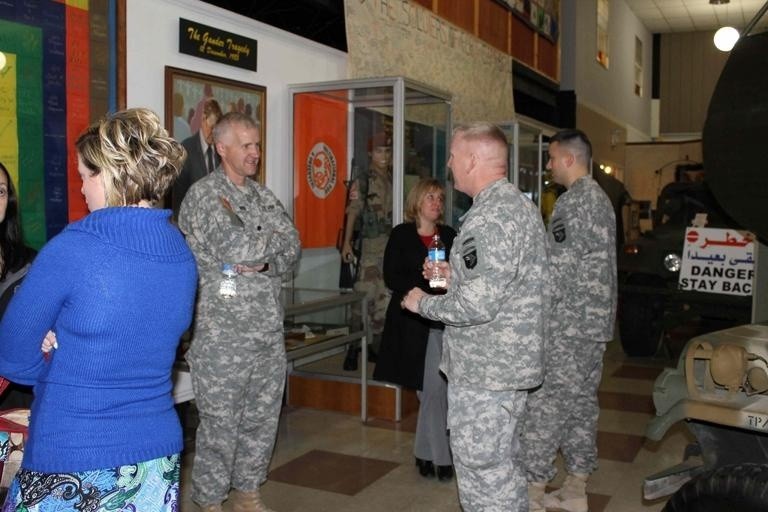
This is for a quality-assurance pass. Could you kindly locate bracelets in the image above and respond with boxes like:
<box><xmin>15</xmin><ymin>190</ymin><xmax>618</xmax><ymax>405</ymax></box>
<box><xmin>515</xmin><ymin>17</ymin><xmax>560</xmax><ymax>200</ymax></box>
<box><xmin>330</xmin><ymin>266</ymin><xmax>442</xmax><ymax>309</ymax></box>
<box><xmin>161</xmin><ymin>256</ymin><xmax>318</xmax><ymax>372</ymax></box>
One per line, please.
<box><xmin>259</xmin><ymin>263</ymin><xmax>269</xmax><ymax>272</ymax></box>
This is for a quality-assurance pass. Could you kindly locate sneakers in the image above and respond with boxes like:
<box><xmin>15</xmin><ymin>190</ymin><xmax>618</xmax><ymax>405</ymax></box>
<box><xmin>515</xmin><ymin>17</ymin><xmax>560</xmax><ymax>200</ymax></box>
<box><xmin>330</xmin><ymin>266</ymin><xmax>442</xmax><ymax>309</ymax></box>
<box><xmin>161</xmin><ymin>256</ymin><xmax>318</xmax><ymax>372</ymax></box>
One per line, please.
<box><xmin>367</xmin><ymin>344</ymin><xmax>377</xmax><ymax>363</ymax></box>
<box><xmin>343</xmin><ymin>345</ymin><xmax>360</xmax><ymax>371</ymax></box>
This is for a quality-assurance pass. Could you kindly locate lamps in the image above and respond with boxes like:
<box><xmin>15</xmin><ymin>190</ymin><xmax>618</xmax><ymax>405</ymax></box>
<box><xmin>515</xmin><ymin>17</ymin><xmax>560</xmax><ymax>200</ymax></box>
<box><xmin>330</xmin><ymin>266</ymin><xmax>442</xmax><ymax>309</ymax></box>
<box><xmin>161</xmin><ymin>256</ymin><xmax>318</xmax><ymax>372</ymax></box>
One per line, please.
<box><xmin>712</xmin><ymin>26</ymin><xmax>740</xmax><ymax>53</ymax></box>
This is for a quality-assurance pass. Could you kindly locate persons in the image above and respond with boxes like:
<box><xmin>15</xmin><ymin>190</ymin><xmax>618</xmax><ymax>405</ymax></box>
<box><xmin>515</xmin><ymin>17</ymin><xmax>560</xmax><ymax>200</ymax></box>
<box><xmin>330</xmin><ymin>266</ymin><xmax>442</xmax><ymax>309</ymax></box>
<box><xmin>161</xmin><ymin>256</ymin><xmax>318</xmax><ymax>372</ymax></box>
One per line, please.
<box><xmin>372</xmin><ymin>180</ymin><xmax>459</xmax><ymax>484</ymax></box>
<box><xmin>164</xmin><ymin>100</ymin><xmax>224</xmax><ymax>221</ymax></box>
<box><xmin>343</xmin><ymin>135</ymin><xmax>393</xmax><ymax>371</ymax></box>
<box><xmin>519</xmin><ymin>128</ymin><xmax>618</xmax><ymax>512</ymax></box>
<box><xmin>399</xmin><ymin>122</ymin><xmax>551</xmax><ymax>511</ymax></box>
<box><xmin>1</xmin><ymin>161</ymin><xmax>39</xmax><ymax>412</ymax></box>
<box><xmin>178</xmin><ymin>112</ymin><xmax>302</xmax><ymax>512</ymax></box>
<box><xmin>0</xmin><ymin>107</ymin><xmax>201</xmax><ymax>512</ymax></box>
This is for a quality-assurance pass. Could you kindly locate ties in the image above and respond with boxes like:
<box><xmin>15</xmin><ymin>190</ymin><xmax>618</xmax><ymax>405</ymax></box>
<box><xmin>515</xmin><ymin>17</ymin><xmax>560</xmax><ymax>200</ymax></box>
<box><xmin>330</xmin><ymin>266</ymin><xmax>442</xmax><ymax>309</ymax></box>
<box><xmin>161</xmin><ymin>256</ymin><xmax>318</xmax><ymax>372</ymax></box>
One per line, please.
<box><xmin>207</xmin><ymin>147</ymin><xmax>214</xmax><ymax>174</ymax></box>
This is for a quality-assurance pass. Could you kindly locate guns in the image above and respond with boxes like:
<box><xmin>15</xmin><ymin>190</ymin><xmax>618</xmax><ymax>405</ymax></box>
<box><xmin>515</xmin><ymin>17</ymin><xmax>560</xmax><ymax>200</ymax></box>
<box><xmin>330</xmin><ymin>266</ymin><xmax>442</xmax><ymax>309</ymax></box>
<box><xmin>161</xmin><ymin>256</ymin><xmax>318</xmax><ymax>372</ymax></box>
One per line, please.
<box><xmin>336</xmin><ymin>158</ymin><xmax>361</xmax><ymax>288</ymax></box>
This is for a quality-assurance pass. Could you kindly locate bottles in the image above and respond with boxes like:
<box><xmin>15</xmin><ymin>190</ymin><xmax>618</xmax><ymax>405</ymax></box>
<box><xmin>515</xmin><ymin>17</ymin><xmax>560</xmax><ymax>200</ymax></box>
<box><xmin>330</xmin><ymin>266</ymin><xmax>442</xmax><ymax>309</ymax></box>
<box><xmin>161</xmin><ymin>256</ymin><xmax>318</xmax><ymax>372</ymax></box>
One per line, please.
<box><xmin>218</xmin><ymin>264</ymin><xmax>238</xmax><ymax>299</ymax></box>
<box><xmin>427</xmin><ymin>233</ymin><xmax>448</xmax><ymax>289</ymax></box>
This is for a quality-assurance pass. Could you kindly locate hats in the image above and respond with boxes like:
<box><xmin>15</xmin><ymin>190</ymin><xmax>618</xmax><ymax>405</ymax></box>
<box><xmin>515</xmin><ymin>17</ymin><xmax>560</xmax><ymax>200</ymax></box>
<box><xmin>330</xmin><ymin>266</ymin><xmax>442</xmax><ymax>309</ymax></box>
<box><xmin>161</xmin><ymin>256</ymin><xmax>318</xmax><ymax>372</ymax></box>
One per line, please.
<box><xmin>368</xmin><ymin>133</ymin><xmax>392</xmax><ymax>151</ymax></box>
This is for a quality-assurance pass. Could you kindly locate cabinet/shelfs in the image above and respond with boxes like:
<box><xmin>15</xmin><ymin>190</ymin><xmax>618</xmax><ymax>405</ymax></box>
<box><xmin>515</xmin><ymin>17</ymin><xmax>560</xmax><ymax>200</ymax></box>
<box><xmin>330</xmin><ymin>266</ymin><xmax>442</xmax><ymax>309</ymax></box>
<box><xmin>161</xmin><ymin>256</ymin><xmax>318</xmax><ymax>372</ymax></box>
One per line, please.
<box><xmin>174</xmin><ymin>284</ymin><xmax>367</xmax><ymax>425</ymax></box>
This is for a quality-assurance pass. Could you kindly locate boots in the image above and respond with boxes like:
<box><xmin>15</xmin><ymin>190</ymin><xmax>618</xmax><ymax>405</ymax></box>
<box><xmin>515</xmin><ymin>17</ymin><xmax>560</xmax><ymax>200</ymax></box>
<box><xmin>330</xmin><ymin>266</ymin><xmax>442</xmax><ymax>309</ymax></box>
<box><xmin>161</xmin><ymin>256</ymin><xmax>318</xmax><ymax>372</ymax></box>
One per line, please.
<box><xmin>527</xmin><ymin>471</ymin><xmax>589</xmax><ymax>512</ymax></box>
<box><xmin>230</xmin><ymin>488</ymin><xmax>272</xmax><ymax>511</ymax></box>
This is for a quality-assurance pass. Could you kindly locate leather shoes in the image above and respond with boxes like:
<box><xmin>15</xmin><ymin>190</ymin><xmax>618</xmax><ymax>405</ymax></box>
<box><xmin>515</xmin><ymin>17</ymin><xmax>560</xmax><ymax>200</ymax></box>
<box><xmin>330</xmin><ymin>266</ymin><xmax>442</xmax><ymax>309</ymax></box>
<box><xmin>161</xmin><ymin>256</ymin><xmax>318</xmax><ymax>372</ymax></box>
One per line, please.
<box><xmin>437</xmin><ymin>466</ymin><xmax>452</xmax><ymax>484</ymax></box>
<box><xmin>414</xmin><ymin>456</ymin><xmax>435</xmax><ymax>478</ymax></box>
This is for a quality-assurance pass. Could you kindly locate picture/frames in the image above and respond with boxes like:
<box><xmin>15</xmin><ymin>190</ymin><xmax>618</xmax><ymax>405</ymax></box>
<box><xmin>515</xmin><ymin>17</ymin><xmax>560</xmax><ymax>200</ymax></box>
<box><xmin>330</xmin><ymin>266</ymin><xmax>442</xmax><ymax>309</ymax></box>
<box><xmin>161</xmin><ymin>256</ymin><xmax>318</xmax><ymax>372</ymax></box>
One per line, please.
<box><xmin>165</xmin><ymin>65</ymin><xmax>268</xmax><ymax>214</ymax></box>
